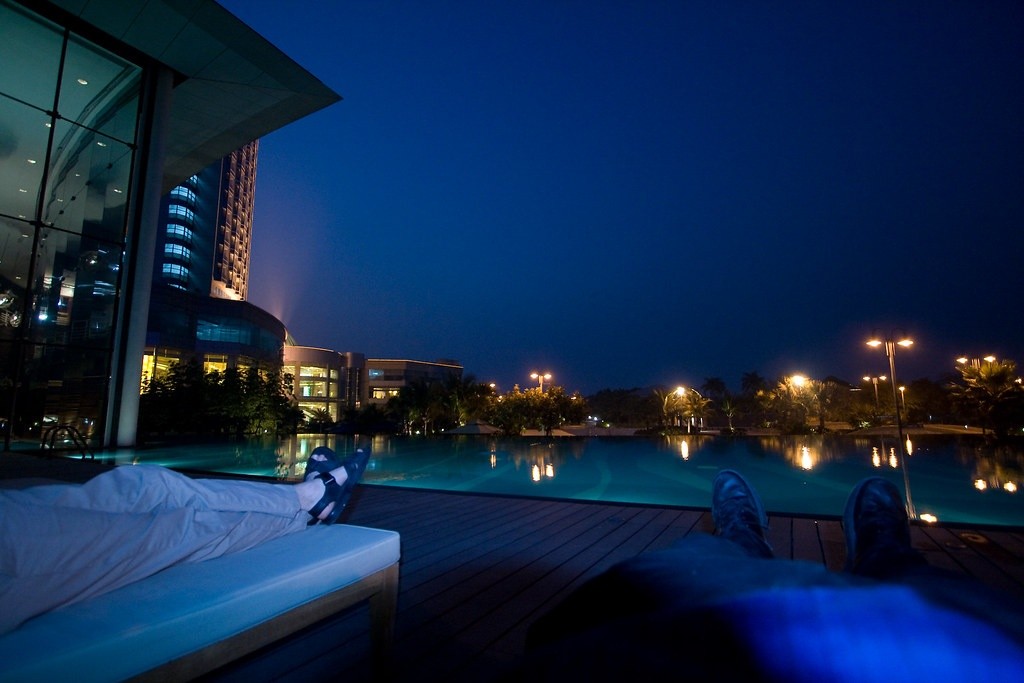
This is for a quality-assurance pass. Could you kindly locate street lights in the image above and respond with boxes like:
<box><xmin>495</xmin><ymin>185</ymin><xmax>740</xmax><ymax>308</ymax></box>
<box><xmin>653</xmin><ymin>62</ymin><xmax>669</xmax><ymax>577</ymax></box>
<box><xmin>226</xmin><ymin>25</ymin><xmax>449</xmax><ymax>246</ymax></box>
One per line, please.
<box><xmin>956</xmin><ymin>351</ymin><xmax>997</xmax><ymax>434</ymax></box>
<box><xmin>864</xmin><ymin>372</ymin><xmax>887</xmax><ymax>415</ymax></box>
<box><xmin>530</xmin><ymin>369</ymin><xmax>552</xmax><ymax>393</ymax></box>
<box><xmin>899</xmin><ymin>384</ymin><xmax>906</xmax><ymax>414</ymax></box>
<box><xmin>865</xmin><ymin>325</ymin><xmax>916</xmax><ymax>517</ymax></box>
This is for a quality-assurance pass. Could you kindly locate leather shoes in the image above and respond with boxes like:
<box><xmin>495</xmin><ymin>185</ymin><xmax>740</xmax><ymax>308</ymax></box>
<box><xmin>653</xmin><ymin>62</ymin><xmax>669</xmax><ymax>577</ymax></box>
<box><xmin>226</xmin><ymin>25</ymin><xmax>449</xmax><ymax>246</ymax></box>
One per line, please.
<box><xmin>842</xmin><ymin>477</ymin><xmax>913</xmax><ymax>585</ymax></box>
<box><xmin>709</xmin><ymin>469</ymin><xmax>775</xmax><ymax>561</ymax></box>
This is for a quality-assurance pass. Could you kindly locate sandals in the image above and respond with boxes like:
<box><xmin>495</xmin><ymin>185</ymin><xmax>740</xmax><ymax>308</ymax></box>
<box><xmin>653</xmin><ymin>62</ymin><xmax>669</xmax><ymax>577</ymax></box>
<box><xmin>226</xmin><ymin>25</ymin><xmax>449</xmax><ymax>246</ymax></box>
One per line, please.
<box><xmin>307</xmin><ymin>445</ymin><xmax>372</xmax><ymax>526</ymax></box>
<box><xmin>304</xmin><ymin>447</ymin><xmax>340</xmax><ymax>526</ymax></box>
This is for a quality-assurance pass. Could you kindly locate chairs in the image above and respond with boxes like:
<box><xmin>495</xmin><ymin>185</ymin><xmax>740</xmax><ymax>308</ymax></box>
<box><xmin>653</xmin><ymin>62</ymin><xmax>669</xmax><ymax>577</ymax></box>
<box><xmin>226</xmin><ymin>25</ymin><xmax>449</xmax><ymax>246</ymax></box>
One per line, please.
<box><xmin>0</xmin><ymin>523</ymin><xmax>401</xmax><ymax>683</ymax></box>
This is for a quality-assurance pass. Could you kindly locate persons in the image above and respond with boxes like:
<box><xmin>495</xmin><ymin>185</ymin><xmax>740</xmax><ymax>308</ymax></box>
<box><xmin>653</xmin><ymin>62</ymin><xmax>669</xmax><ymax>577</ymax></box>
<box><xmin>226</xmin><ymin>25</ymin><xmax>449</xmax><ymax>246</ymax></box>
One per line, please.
<box><xmin>454</xmin><ymin>469</ymin><xmax>1023</xmax><ymax>683</ymax></box>
<box><xmin>0</xmin><ymin>437</ymin><xmax>371</xmax><ymax>638</ymax></box>
